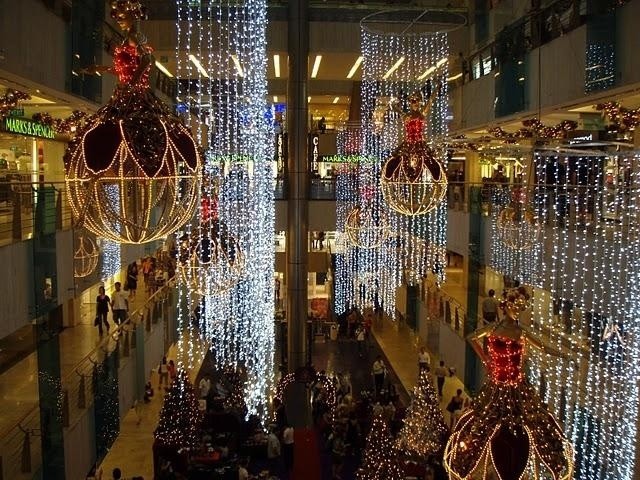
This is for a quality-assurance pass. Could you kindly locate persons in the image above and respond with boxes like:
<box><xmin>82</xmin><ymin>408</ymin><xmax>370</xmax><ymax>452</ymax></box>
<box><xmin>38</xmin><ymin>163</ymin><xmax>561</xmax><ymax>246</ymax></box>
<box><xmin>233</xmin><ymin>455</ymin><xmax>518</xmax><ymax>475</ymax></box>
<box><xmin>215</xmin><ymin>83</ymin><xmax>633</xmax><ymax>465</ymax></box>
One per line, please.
<box><xmin>446</xmin><ymin>388</ymin><xmax>463</xmax><ymax>428</ymax></box>
<box><xmin>434</xmin><ymin>360</ymin><xmax>449</xmax><ymax>396</ymax></box>
<box><xmin>318</xmin><ymin>116</ymin><xmax>327</xmax><ymax>134</ymax></box>
<box><xmin>492</xmin><ymin>165</ymin><xmax>510</xmax><ymax>210</ymax></box>
<box><xmin>372</xmin><ymin>355</ymin><xmax>387</xmax><ymax>397</ymax></box>
<box><xmin>481</xmin><ymin>288</ymin><xmax>501</xmax><ymax>356</ymax></box>
<box><xmin>235</xmin><ymin>370</ymin><xmax>372</xmax><ymax>480</ymax></box>
<box><xmin>447</xmin><ymin>367</ymin><xmax>457</xmax><ymax>377</ymax></box>
<box><xmin>148</xmin><ymin>250</ymin><xmax>169</xmax><ymax>298</ymax></box>
<box><xmin>355</xmin><ymin>323</ymin><xmax>367</xmax><ymax>359</ymax></box>
<box><xmin>602</xmin><ymin>319</ymin><xmax>627</xmax><ymax>365</ymax></box>
<box><xmin>347</xmin><ymin>310</ymin><xmax>357</xmax><ymax>343</ymax></box>
<box><xmin>417</xmin><ymin>346</ymin><xmax>431</xmax><ymax>376</ymax></box>
<box><xmin>370</xmin><ymin>279</ymin><xmax>384</xmax><ymax>314</ymax></box>
<box><xmin>418</xmin><ymin>256</ymin><xmax>430</xmax><ymax>287</ymax></box>
<box><xmin>562</xmin><ymin>299</ymin><xmax>572</xmax><ymax>335</ymax></box>
<box><xmin>274</xmin><ymin>279</ymin><xmax>280</xmax><ymax>298</ymax></box>
<box><xmin>372</xmin><ymin>401</ymin><xmax>385</xmax><ymax>419</ymax></box>
<box><xmin>44</xmin><ymin>283</ymin><xmax>52</xmax><ymax>300</ymax></box>
<box><xmin>92</xmin><ymin>247</ymin><xmax>191</xmax><ymax>334</ymax></box>
<box><xmin>552</xmin><ymin>297</ymin><xmax>560</xmax><ymax>327</ymax></box>
<box><xmin>365</xmin><ymin>314</ymin><xmax>373</xmax><ymax>335</ymax></box>
<box><xmin>112</xmin><ymin>468</ymin><xmax>121</xmax><ymax>480</ymax></box>
<box><xmin>198</xmin><ymin>373</ymin><xmax>213</xmax><ymax>412</ymax></box>
<box><xmin>133</xmin><ymin>357</ymin><xmax>178</xmax><ymax>423</ymax></box>
<box><xmin>432</xmin><ymin>254</ymin><xmax>447</xmax><ymax>291</ymax></box>
<box><xmin>382</xmin><ymin>399</ymin><xmax>397</xmax><ymax>433</ymax></box>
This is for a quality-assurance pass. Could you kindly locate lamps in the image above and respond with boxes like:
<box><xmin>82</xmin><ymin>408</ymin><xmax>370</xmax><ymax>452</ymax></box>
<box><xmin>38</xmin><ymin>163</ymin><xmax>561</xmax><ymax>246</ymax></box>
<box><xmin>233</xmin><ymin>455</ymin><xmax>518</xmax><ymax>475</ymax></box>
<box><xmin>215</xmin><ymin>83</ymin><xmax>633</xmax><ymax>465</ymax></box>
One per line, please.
<box><xmin>496</xmin><ymin>174</ymin><xmax>540</xmax><ymax>251</ymax></box>
<box><xmin>65</xmin><ymin>0</ymin><xmax>202</xmax><ymax>244</ymax></box>
<box><xmin>443</xmin><ymin>280</ymin><xmax>574</xmax><ymax>480</ymax></box>
<box><xmin>382</xmin><ymin>18</ymin><xmax>447</xmax><ymax>216</ymax></box>
<box><xmin>176</xmin><ymin>178</ymin><xmax>243</xmax><ymax>295</ymax></box>
<box><xmin>348</xmin><ymin>205</ymin><xmax>388</xmax><ymax>249</ymax></box>
